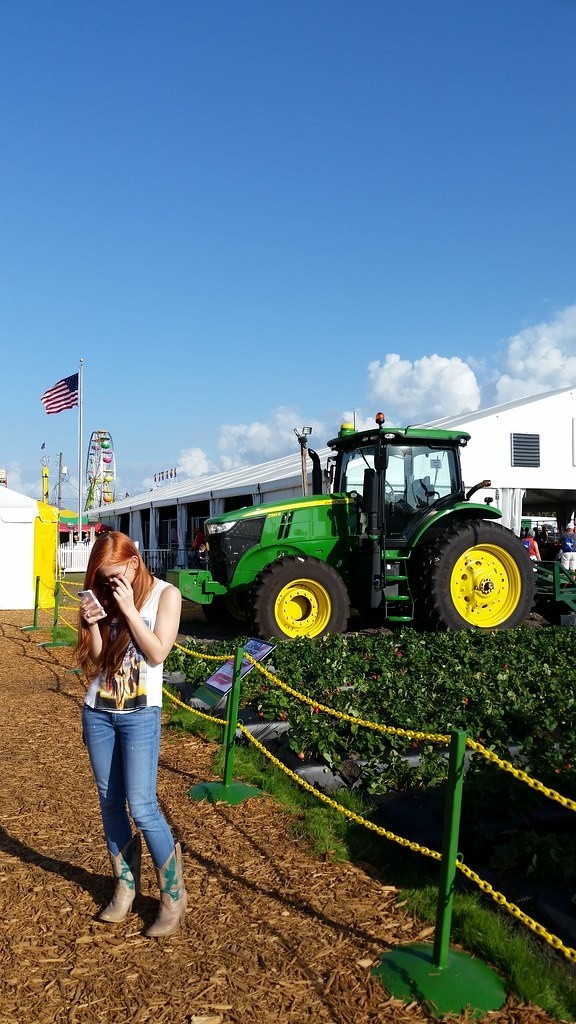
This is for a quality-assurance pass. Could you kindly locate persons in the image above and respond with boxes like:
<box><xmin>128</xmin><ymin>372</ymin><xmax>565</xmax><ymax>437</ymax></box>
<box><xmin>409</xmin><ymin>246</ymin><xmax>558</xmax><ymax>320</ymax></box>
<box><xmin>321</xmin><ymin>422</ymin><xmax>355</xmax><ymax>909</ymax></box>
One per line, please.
<box><xmin>192</xmin><ymin>528</ymin><xmax>206</xmax><ymax>569</ymax></box>
<box><xmin>73</xmin><ymin>530</ymin><xmax>189</xmax><ymax>940</ymax></box>
<box><xmin>520</xmin><ymin>526</ymin><xmax>549</xmax><ymax>572</ymax></box>
<box><xmin>557</xmin><ymin>523</ymin><xmax>576</xmax><ymax>581</ymax></box>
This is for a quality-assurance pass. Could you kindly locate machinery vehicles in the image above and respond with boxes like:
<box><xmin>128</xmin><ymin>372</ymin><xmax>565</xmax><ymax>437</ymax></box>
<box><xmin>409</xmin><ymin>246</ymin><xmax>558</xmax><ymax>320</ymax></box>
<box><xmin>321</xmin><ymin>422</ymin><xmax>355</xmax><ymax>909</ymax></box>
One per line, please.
<box><xmin>165</xmin><ymin>412</ymin><xmax>576</xmax><ymax>640</ymax></box>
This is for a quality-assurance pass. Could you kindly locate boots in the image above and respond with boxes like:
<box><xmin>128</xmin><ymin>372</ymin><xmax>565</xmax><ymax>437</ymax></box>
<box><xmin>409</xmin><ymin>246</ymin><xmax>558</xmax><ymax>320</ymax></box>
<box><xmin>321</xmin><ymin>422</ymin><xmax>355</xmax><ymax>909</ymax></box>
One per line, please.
<box><xmin>144</xmin><ymin>841</ymin><xmax>188</xmax><ymax>937</ymax></box>
<box><xmin>97</xmin><ymin>830</ymin><xmax>142</xmax><ymax>923</ymax></box>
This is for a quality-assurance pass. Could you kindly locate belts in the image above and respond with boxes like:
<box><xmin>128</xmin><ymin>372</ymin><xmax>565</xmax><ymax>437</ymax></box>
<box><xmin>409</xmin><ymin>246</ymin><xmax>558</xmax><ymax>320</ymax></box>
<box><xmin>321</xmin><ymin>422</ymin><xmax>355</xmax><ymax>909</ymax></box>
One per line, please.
<box><xmin>563</xmin><ymin>551</ymin><xmax>576</xmax><ymax>552</ymax></box>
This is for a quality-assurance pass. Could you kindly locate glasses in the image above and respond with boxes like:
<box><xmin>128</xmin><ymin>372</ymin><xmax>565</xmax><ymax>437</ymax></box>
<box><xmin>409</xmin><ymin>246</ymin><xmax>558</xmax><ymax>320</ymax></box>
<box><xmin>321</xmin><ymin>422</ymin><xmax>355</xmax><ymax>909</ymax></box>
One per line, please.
<box><xmin>101</xmin><ymin>559</ymin><xmax>131</xmax><ymax>587</ymax></box>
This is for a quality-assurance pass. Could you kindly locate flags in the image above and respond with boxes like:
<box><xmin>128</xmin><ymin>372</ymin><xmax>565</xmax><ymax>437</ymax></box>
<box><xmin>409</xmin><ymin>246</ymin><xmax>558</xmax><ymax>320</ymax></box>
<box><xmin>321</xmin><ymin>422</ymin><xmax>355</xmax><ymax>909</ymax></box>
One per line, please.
<box><xmin>40</xmin><ymin>441</ymin><xmax>46</xmax><ymax>451</ymax></box>
<box><xmin>39</xmin><ymin>371</ymin><xmax>80</xmax><ymax>415</ymax></box>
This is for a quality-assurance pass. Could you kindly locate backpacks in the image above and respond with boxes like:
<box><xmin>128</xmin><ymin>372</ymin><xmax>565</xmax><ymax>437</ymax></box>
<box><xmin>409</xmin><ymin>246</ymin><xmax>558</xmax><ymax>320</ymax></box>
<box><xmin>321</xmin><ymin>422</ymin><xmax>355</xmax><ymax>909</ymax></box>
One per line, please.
<box><xmin>564</xmin><ymin>532</ymin><xmax>575</xmax><ymax>551</ymax></box>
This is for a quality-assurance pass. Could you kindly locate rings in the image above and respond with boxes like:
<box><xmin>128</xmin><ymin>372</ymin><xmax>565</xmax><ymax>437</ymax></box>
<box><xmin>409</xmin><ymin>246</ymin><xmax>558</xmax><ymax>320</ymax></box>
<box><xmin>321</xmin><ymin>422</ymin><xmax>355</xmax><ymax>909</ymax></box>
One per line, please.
<box><xmin>87</xmin><ymin>613</ymin><xmax>91</xmax><ymax>619</ymax></box>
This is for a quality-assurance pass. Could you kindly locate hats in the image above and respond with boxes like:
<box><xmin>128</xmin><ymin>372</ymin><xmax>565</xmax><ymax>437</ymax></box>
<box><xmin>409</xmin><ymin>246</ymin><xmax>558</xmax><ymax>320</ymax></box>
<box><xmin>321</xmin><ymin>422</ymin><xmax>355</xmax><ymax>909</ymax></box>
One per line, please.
<box><xmin>566</xmin><ymin>523</ymin><xmax>575</xmax><ymax>529</ymax></box>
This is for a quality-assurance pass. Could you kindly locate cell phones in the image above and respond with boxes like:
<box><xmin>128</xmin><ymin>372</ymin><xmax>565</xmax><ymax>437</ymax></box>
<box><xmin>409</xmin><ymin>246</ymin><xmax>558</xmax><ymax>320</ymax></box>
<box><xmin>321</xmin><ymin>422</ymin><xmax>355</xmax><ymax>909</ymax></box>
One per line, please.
<box><xmin>78</xmin><ymin>589</ymin><xmax>106</xmax><ymax>616</ymax></box>
